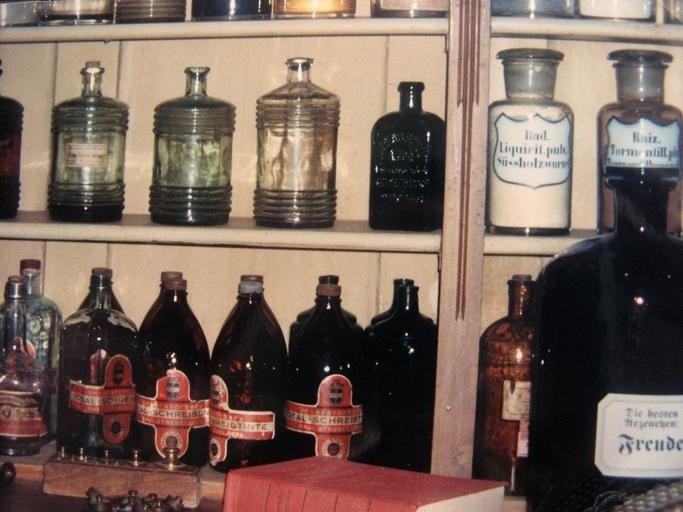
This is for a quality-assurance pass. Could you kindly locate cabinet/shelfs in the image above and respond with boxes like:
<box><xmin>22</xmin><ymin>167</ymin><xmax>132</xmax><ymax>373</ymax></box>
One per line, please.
<box><xmin>0</xmin><ymin>0</ymin><xmax>683</xmax><ymax>512</ymax></box>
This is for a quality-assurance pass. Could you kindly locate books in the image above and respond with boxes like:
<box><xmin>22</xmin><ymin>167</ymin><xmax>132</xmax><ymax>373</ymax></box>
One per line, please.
<box><xmin>221</xmin><ymin>455</ymin><xmax>509</xmax><ymax>512</ymax></box>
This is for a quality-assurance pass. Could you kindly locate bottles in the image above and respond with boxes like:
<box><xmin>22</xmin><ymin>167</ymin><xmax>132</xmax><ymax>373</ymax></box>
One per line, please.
<box><xmin>0</xmin><ymin>0</ymin><xmax>683</xmax><ymax>512</ymax></box>
